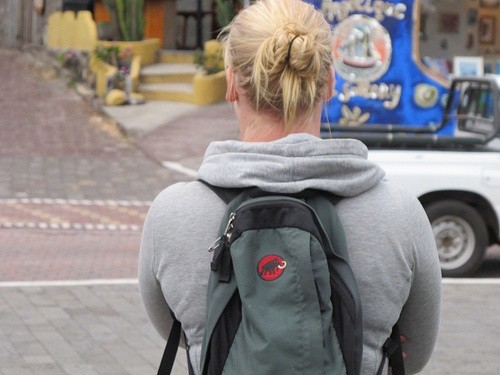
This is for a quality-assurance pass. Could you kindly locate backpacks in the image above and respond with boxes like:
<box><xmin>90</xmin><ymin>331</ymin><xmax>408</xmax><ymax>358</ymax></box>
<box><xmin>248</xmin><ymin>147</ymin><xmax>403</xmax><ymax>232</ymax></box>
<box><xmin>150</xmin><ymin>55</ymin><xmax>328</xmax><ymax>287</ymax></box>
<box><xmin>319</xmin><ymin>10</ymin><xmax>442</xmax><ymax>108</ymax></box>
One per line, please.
<box><xmin>195</xmin><ymin>177</ymin><xmax>361</xmax><ymax>375</ymax></box>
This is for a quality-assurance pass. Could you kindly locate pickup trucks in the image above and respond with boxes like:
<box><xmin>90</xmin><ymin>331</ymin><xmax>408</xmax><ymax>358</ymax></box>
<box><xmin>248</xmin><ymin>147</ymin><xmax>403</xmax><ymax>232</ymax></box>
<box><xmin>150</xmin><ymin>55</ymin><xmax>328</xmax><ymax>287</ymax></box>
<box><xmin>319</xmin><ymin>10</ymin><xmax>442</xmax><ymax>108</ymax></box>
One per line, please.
<box><xmin>322</xmin><ymin>79</ymin><xmax>499</xmax><ymax>278</ymax></box>
